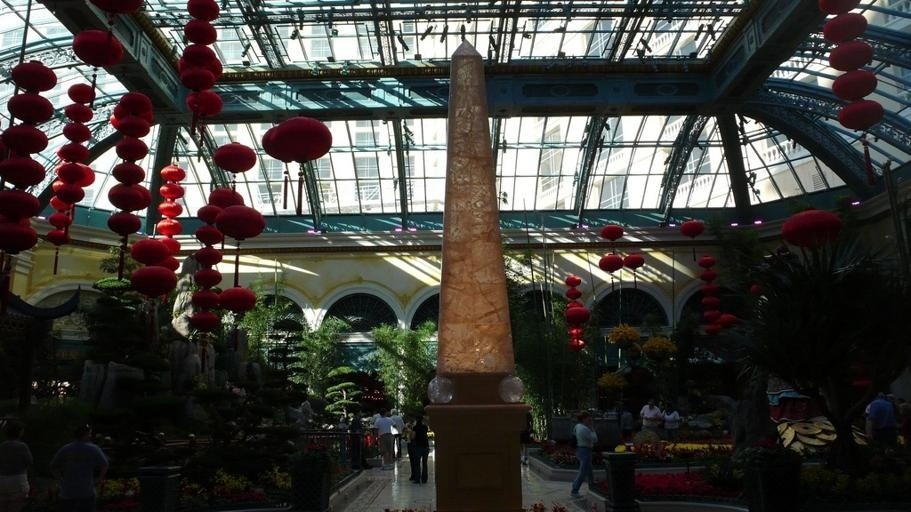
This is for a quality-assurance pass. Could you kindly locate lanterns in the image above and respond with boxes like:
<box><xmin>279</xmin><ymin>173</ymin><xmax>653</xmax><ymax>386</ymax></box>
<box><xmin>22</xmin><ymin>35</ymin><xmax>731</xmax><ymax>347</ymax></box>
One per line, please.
<box><xmin>558</xmin><ymin>210</ymin><xmax>842</xmax><ymax>369</ymax></box>
<box><xmin>813</xmin><ymin>0</ymin><xmax>886</xmax><ymax>134</ymax></box>
<box><xmin>1</xmin><ymin>1</ymin><xmax>334</xmax><ymax>337</ymax></box>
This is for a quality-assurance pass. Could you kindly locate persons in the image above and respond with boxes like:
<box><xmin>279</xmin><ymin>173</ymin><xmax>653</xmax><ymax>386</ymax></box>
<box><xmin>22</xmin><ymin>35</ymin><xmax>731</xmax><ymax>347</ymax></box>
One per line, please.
<box><xmin>520</xmin><ymin>391</ymin><xmax>911</xmax><ymax>512</ymax></box>
<box><xmin>2</xmin><ymin>391</ymin><xmax>429</xmax><ymax>512</ymax></box>
